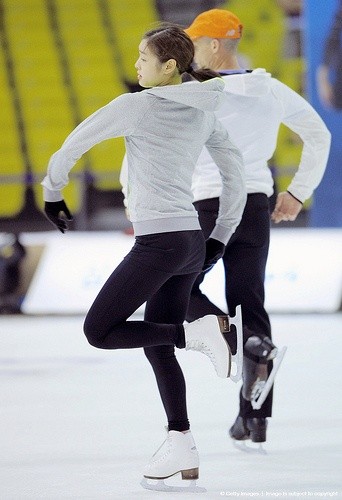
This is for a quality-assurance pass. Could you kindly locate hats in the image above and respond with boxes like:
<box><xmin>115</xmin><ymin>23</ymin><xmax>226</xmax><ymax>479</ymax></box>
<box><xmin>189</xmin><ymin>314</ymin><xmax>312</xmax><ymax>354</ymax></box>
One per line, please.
<box><xmin>183</xmin><ymin>9</ymin><xmax>244</xmax><ymax>41</ymax></box>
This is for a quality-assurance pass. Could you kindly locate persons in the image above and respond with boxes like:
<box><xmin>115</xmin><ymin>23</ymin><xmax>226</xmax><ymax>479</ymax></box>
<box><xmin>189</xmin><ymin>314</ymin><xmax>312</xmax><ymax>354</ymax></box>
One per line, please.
<box><xmin>180</xmin><ymin>8</ymin><xmax>332</xmax><ymax>455</ymax></box>
<box><xmin>40</xmin><ymin>24</ymin><xmax>243</xmax><ymax>489</ymax></box>
<box><xmin>317</xmin><ymin>0</ymin><xmax>342</xmax><ymax>115</ymax></box>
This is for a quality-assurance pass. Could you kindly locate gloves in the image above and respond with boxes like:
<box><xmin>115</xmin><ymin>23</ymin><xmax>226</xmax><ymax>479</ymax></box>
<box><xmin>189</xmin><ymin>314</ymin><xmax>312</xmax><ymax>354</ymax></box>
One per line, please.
<box><xmin>42</xmin><ymin>199</ymin><xmax>74</xmax><ymax>234</ymax></box>
<box><xmin>202</xmin><ymin>236</ymin><xmax>226</xmax><ymax>274</ymax></box>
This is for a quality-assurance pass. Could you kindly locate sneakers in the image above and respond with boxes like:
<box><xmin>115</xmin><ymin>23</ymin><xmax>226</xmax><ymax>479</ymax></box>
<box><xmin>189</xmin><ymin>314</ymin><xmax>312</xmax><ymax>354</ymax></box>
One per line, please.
<box><xmin>141</xmin><ymin>428</ymin><xmax>199</xmax><ymax>481</ymax></box>
<box><xmin>182</xmin><ymin>313</ymin><xmax>235</xmax><ymax>380</ymax></box>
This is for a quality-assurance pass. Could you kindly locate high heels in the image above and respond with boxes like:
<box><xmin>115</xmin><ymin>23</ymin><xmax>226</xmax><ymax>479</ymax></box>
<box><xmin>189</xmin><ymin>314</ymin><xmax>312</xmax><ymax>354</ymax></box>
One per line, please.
<box><xmin>230</xmin><ymin>413</ymin><xmax>269</xmax><ymax>443</ymax></box>
<box><xmin>241</xmin><ymin>331</ymin><xmax>277</xmax><ymax>402</ymax></box>
